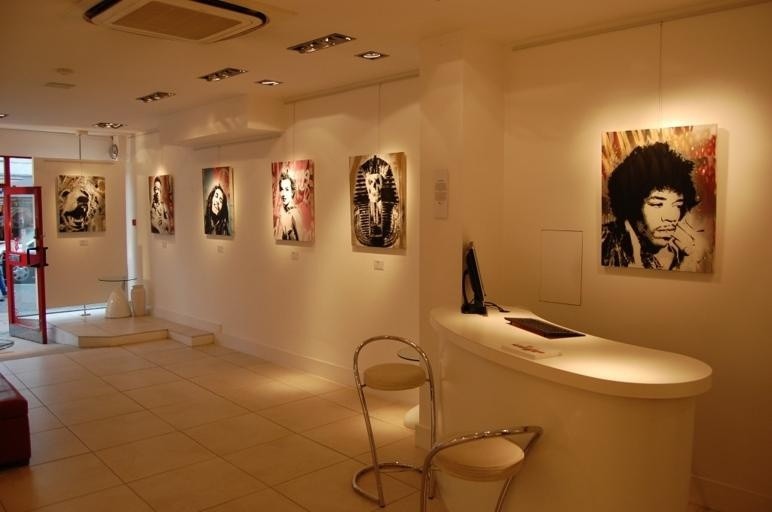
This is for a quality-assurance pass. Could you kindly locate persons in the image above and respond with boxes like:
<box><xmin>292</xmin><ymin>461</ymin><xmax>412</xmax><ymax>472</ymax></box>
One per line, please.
<box><xmin>149</xmin><ymin>176</ymin><xmax>174</xmax><ymax>232</ymax></box>
<box><xmin>10</xmin><ymin>234</ymin><xmax>19</xmax><ymax>254</ymax></box>
<box><xmin>0</xmin><ymin>212</ymin><xmax>8</xmax><ymax>302</ymax></box>
<box><xmin>204</xmin><ymin>184</ymin><xmax>230</xmax><ymax>235</ymax></box>
<box><xmin>601</xmin><ymin>141</ymin><xmax>711</xmax><ymax>273</ymax></box>
<box><xmin>271</xmin><ymin>173</ymin><xmax>304</xmax><ymax>242</ymax></box>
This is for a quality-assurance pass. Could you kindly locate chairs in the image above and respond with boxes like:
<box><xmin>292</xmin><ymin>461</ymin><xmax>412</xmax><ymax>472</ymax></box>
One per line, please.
<box><xmin>417</xmin><ymin>426</ymin><xmax>546</xmax><ymax>512</ymax></box>
<box><xmin>351</xmin><ymin>333</ymin><xmax>436</xmax><ymax>508</ymax></box>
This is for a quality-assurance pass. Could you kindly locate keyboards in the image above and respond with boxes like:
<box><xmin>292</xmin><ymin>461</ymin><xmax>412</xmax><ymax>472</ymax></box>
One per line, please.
<box><xmin>506</xmin><ymin>318</ymin><xmax>581</xmax><ymax>337</ymax></box>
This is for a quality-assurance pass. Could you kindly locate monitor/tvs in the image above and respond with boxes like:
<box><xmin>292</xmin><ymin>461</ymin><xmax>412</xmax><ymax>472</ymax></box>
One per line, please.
<box><xmin>461</xmin><ymin>240</ymin><xmax>487</xmax><ymax>314</ymax></box>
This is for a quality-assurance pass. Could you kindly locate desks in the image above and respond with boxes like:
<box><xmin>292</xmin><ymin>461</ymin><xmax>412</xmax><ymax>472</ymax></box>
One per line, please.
<box><xmin>427</xmin><ymin>302</ymin><xmax>714</xmax><ymax>511</ymax></box>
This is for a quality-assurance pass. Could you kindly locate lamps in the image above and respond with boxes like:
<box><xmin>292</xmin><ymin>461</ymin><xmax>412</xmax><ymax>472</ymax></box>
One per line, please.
<box><xmin>81</xmin><ymin>1</ymin><xmax>270</xmax><ymax>43</ymax></box>
